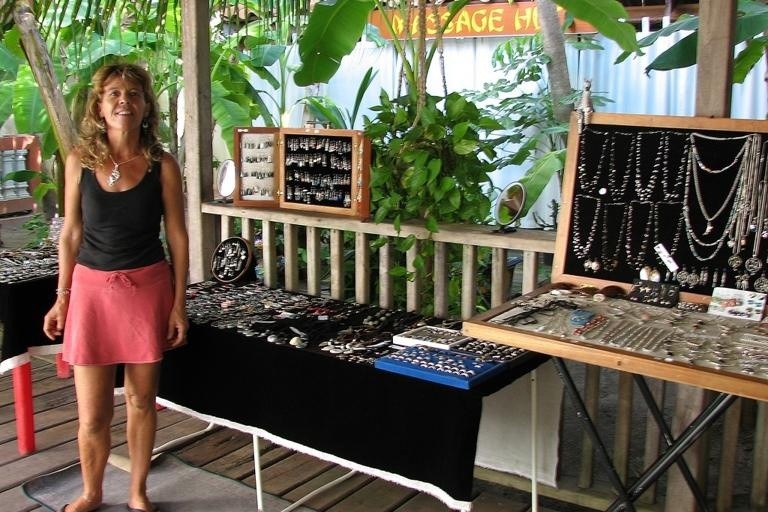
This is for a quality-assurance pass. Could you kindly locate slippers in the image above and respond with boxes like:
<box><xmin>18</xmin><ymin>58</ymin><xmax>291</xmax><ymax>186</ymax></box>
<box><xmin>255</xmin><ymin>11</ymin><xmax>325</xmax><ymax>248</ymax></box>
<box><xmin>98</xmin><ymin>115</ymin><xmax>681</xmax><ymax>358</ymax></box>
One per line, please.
<box><xmin>59</xmin><ymin>504</ymin><xmax>103</xmax><ymax>512</ymax></box>
<box><xmin>126</xmin><ymin>503</ymin><xmax>160</xmax><ymax>512</ymax></box>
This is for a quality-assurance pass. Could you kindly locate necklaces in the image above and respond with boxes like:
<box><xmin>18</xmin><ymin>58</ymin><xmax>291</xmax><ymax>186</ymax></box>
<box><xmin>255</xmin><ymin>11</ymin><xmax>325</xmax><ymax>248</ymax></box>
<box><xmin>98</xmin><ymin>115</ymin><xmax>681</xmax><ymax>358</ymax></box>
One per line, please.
<box><xmin>107</xmin><ymin>153</ymin><xmax>150</xmax><ymax>185</ymax></box>
<box><xmin>526</xmin><ymin>125</ymin><xmax>767</xmax><ymax>381</ymax></box>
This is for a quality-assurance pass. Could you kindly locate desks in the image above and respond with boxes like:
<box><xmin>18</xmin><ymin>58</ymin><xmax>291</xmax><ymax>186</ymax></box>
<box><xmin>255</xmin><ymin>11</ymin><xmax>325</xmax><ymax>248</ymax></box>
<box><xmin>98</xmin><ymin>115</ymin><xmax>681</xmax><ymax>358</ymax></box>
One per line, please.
<box><xmin>114</xmin><ymin>277</ymin><xmax>553</xmax><ymax>512</ymax></box>
<box><xmin>0</xmin><ymin>253</ymin><xmax>65</xmax><ymax>454</ymax></box>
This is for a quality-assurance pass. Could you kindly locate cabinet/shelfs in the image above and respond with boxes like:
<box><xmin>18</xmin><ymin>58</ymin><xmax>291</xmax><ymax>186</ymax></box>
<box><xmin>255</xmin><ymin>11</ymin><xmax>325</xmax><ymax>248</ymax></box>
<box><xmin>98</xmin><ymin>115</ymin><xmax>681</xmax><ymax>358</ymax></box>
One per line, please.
<box><xmin>462</xmin><ymin>107</ymin><xmax>768</xmax><ymax>512</ymax></box>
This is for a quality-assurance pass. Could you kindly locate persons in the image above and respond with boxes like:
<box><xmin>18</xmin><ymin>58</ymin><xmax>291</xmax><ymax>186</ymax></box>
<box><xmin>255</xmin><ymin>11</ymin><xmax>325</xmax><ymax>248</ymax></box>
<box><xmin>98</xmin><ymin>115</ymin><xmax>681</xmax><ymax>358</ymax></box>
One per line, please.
<box><xmin>43</xmin><ymin>63</ymin><xmax>189</xmax><ymax>512</ymax></box>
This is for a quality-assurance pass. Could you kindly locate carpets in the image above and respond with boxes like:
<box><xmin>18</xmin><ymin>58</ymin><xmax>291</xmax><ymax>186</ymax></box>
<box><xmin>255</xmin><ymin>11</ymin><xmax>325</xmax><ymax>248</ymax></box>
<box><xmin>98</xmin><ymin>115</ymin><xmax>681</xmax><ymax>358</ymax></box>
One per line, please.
<box><xmin>24</xmin><ymin>452</ymin><xmax>340</xmax><ymax>512</ymax></box>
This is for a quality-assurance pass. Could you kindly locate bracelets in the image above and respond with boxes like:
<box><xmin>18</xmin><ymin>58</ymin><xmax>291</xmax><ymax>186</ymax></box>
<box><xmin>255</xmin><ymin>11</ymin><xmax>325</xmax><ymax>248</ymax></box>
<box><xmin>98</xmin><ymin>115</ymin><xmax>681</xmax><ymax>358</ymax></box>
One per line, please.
<box><xmin>56</xmin><ymin>288</ymin><xmax>71</xmax><ymax>295</ymax></box>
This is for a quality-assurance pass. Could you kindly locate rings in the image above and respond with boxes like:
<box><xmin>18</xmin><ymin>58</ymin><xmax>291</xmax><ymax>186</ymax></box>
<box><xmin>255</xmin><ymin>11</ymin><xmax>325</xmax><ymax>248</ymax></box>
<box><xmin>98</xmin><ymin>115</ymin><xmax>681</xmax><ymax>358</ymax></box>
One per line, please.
<box><xmin>386</xmin><ymin>321</ymin><xmax>525</xmax><ymax>379</ymax></box>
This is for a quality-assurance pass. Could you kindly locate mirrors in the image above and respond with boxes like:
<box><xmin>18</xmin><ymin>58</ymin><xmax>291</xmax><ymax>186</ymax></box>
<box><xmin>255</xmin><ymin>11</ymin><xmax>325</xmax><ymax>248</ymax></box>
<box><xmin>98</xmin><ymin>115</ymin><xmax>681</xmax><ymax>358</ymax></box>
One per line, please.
<box><xmin>492</xmin><ymin>182</ymin><xmax>526</xmax><ymax>233</ymax></box>
